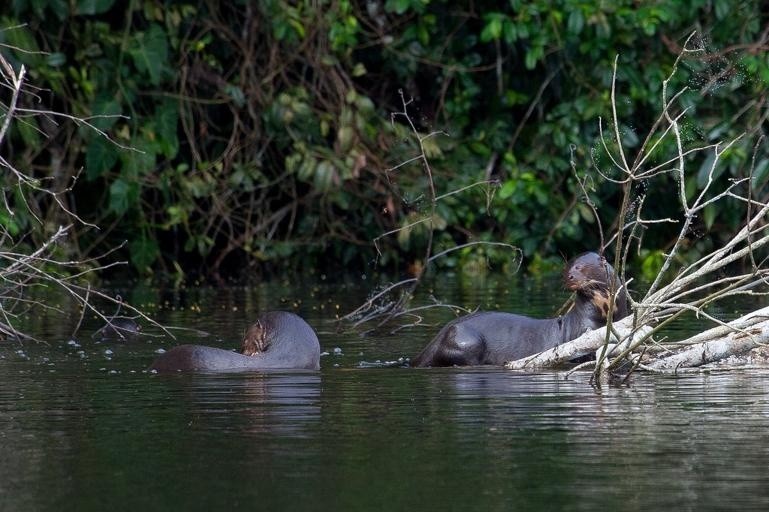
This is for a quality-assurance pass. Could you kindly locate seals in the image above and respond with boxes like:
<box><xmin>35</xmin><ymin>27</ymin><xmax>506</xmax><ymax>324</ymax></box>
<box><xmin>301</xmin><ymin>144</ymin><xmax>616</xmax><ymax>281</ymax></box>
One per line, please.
<box><xmin>405</xmin><ymin>251</ymin><xmax>630</xmax><ymax>369</ymax></box>
<box><xmin>146</xmin><ymin>310</ymin><xmax>322</xmax><ymax>376</ymax></box>
